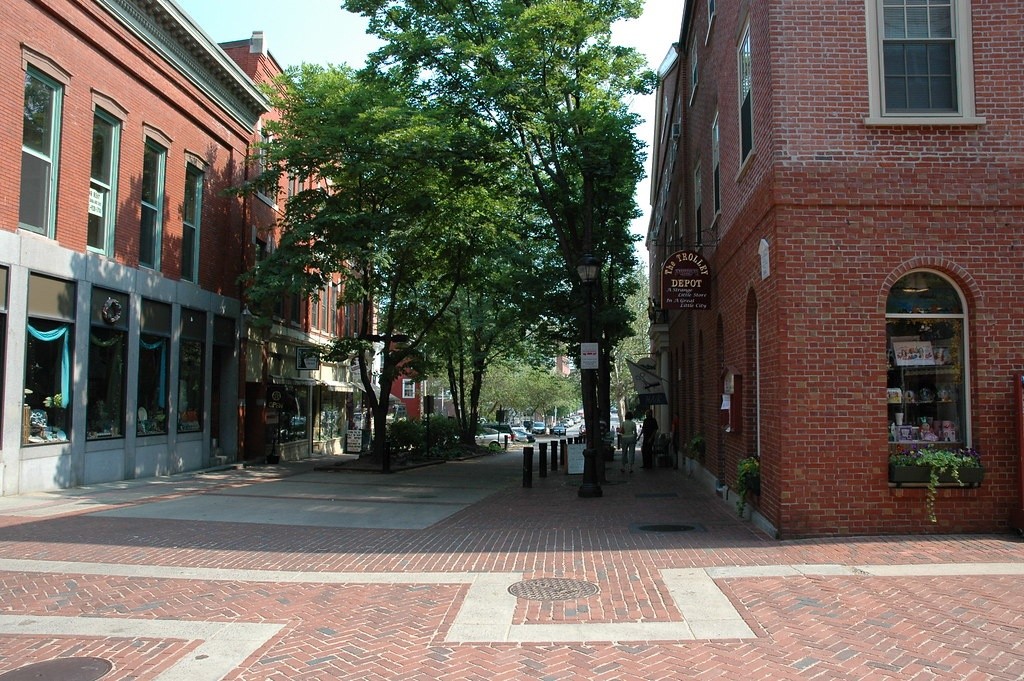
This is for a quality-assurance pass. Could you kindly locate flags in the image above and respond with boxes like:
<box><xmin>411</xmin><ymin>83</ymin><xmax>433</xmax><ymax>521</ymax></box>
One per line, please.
<box><xmin>626</xmin><ymin>360</ymin><xmax>667</xmax><ymax>405</ymax></box>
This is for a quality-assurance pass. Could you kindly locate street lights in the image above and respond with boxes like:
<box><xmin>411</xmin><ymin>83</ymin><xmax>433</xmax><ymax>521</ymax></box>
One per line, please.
<box><xmin>574</xmin><ymin>251</ymin><xmax>605</xmax><ymax>499</ymax></box>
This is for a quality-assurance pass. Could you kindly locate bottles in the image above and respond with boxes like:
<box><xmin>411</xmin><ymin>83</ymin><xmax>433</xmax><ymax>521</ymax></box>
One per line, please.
<box><xmin>138</xmin><ymin>407</ymin><xmax>147</xmax><ymax>421</ymax></box>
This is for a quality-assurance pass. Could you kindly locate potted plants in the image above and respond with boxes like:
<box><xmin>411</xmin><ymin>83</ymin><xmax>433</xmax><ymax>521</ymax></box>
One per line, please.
<box><xmin>690</xmin><ymin>434</ymin><xmax>705</xmax><ymax>453</ymax></box>
<box><xmin>655</xmin><ymin>433</ymin><xmax>671</xmax><ymax>469</ymax></box>
<box><xmin>266</xmin><ymin>423</ymin><xmax>280</xmax><ymax>464</ymax></box>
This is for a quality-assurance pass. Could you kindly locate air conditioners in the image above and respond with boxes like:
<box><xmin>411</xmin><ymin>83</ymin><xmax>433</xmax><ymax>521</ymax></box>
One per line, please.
<box><xmin>649</xmin><ymin>231</ymin><xmax>657</xmax><ymax>240</ymax></box>
<box><xmin>671</xmin><ymin>122</ymin><xmax>681</xmax><ymax>140</ymax></box>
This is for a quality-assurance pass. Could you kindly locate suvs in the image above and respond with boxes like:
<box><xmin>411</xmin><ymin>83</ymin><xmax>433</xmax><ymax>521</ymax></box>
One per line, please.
<box><xmin>482</xmin><ymin>424</ymin><xmax>515</xmax><ymax>442</ymax></box>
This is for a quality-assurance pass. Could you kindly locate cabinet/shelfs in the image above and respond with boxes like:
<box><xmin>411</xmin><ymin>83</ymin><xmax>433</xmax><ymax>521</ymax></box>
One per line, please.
<box><xmin>887</xmin><ymin>364</ymin><xmax>960</xmax><ymax>441</ymax></box>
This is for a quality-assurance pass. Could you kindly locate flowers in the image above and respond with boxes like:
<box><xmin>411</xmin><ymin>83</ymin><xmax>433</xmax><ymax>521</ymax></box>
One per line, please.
<box><xmin>738</xmin><ymin>456</ymin><xmax>759</xmax><ymax>475</ymax></box>
<box><xmin>887</xmin><ymin>443</ymin><xmax>982</xmax><ymax>466</ymax></box>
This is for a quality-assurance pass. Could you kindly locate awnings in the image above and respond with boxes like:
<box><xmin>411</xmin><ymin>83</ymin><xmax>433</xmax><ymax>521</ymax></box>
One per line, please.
<box><xmin>352</xmin><ymin>381</ymin><xmax>400</xmax><ymax>402</ymax></box>
<box><xmin>321</xmin><ymin>380</ymin><xmax>354</xmax><ymax>392</ymax></box>
<box><xmin>271</xmin><ymin>374</ymin><xmax>316</xmax><ymax>386</ymax></box>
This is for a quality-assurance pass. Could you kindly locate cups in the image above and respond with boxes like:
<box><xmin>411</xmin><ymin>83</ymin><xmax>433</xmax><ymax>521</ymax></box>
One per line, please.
<box><xmin>942</xmin><ymin>421</ymin><xmax>955</xmax><ymax>431</ymax></box>
<box><xmin>895</xmin><ymin>413</ymin><xmax>903</xmax><ymax>426</ymax></box>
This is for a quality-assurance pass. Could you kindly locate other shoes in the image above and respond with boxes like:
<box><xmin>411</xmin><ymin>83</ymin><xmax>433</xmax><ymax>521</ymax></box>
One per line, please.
<box><xmin>640</xmin><ymin>465</ymin><xmax>652</xmax><ymax>469</ymax></box>
<box><xmin>621</xmin><ymin>468</ymin><xmax>624</xmax><ymax>471</ymax></box>
<box><xmin>629</xmin><ymin>470</ymin><xmax>634</xmax><ymax>474</ymax></box>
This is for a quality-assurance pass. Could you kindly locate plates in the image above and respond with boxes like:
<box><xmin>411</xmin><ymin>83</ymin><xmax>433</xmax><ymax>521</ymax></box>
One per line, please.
<box><xmin>57</xmin><ymin>430</ymin><xmax>66</xmax><ymax>441</ymax></box>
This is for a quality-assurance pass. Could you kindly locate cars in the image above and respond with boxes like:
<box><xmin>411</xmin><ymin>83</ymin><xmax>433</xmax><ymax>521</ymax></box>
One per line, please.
<box><xmin>510</xmin><ymin>417</ymin><xmax>535</xmax><ymax>443</ymax></box>
<box><xmin>560</xmin><ymin>408</ymin><xmax>587</xmax><ymax>436</ymax></box>
<box><xmin>531</xmin><ymin>422</ymin><xmax>546</xmax><ymax>434</ymax></box>
<box><xmin>553</xmin><ymin>426</ymin><xmax>566</xmax><ymax>436</ymax></box>
<box><xmin>609</xmin><ymin>406</ymin><xmax>618</xmax><ymax>417</ymax></box>
<box><xmin>474</xmin><ymin>427</ymin><xmax>512</xmax><ymax>449</ymax></box>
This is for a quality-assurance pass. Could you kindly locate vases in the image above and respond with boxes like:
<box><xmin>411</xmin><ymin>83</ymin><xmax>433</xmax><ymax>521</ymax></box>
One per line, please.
<box><xmin>890</xmin><ymin>465</ymin><xmax>987</xmax><ymax>483</ymax></box>
<box><xmin>745</xmin><ymin>474</ymin><xmax>761</xmax><ymax>497</ymax></box>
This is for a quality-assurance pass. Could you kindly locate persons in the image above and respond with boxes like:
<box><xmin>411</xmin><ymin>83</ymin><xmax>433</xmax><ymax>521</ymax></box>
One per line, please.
<box><xmin>671</xmin><ymin>413</ymin><xmax>679</xmax><ymax>468</ymax></box>
<box><xmin>920</xmin><ymin>423</ymin><xmax>938</xmax><ymax>441</ymax></box>
<box><xmin>619</xmin><ymin>412</ymin><xmax>638</xmax><ymax>473</ymax></box>
<box><xmin>611</xmin><ymin>425</ymin><xmax>619</xmax><ymax>435</ymax></box>
<box><xmin>637</xmin><ymin>409</ymin><xmax>658</xmax><ymax>468</ymax></box>
